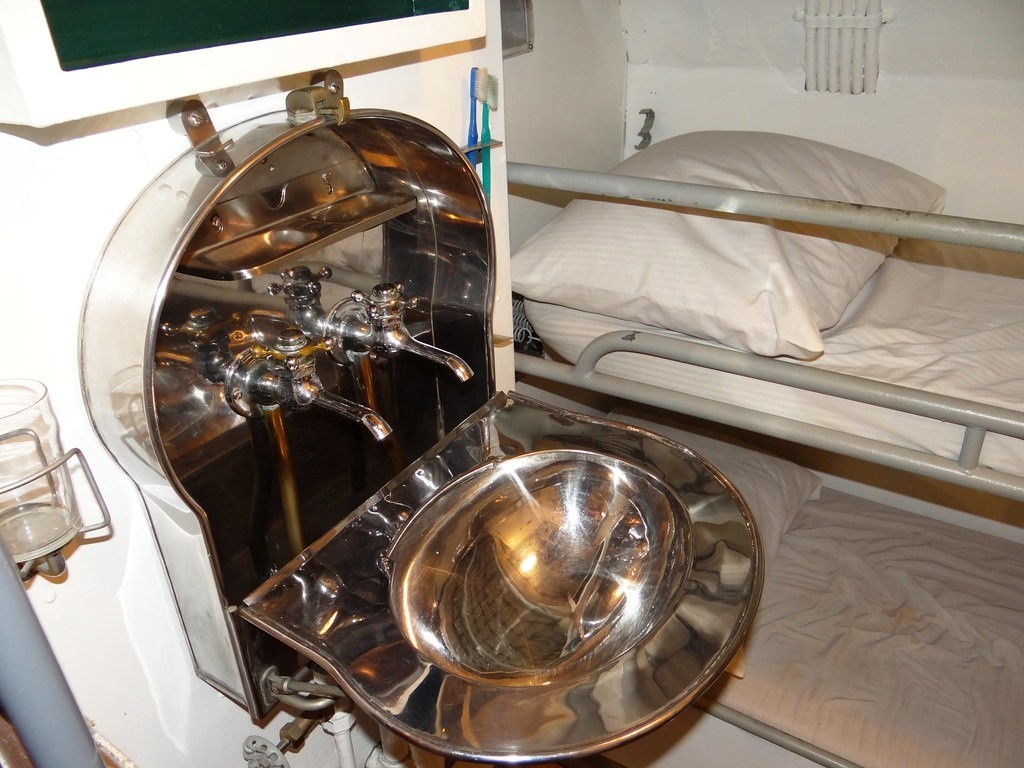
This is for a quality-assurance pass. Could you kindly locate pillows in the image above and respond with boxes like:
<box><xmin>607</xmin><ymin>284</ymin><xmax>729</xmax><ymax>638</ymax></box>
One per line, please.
<box><xmin>509</xmin><ymin>128</ymin><xmax>949</xmax><ymax>363</ymax></box>
<box><xmin>607</xmin><ymin>411</ymin><xmax>822</xmax><ymax>680</ymax></box>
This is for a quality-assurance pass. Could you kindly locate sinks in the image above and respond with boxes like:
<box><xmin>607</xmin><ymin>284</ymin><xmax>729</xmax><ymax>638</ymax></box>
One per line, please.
<box><xmin>387</xmin><ymin>447</ymin><xmax>694</xmax><ymax>688</ymax></box>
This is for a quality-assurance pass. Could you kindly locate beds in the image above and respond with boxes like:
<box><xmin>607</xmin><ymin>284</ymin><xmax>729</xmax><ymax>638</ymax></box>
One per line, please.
<box><xmin>511</xmin><ymin>379</ymin><xmax>1022</xmax><ymax>768</ymax></box>
<box><xmin>525</xmin><ymin>254</ymin><xmax>1022</xmax><ymax>534</ymax></box>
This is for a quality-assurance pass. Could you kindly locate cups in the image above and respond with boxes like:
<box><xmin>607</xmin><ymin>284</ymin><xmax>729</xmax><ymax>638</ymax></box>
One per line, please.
<box><xmin>0</xmin><ymin>379</ymin><xmax>83</xmax><ymax>563</ymax></box>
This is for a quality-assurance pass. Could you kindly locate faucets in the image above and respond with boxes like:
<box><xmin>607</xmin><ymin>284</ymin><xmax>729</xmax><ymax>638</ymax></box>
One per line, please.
<box><xmin>149</xmin><ymin>262</ymin><xmax>351</xmax><ymax>424</ymax></box>
<box><xmin>222</xmin><ymin>326</ymin><xmax>393</xmax><ymax>441</ymax></box>
<box><xmin>322</xmin><ymin>280</ymin><xmax>475</xmax><ymax>383</ymax></box>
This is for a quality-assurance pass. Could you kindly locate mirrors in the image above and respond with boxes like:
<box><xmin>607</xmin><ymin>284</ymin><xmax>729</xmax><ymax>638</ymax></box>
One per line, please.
<box><xmin>499</xmin><ymin>1</ymin><xmax>534</xmax><ymax>59</ymax></box>
